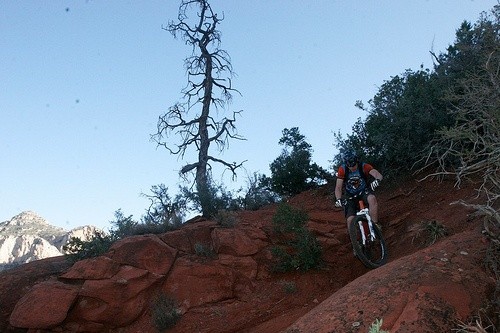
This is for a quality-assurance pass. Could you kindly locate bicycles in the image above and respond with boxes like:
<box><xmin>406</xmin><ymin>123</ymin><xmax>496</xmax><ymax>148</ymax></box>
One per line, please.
<box><xmin>333</xmin><ymin>179</ymin><xmax>388</xmax><ymax>269</ymax></box>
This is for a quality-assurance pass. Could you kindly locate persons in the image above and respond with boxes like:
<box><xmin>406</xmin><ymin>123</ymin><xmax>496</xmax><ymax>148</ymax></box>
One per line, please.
<box><xmin>334</xmin><ymin>156</ymin><xmax>383</xmax><ymax>257</ymax></box>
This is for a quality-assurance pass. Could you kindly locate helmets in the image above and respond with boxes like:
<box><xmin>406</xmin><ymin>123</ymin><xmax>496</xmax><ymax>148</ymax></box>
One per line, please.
<box><xmin>342</xmin><ymin>149</ymin><xmax>358</xmax><ymax>167</ymax></box>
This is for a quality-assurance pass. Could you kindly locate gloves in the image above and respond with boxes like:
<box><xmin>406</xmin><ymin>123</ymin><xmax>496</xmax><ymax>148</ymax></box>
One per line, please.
<box><xmin>370</xmin><ymin>178</ymin><xmax>381</xmax><ymax>192</ymax></box>
<box><xmin>334</xmin><ymin>197</ymin><xmax>347</xmax><ymax>208</ymax></box>
